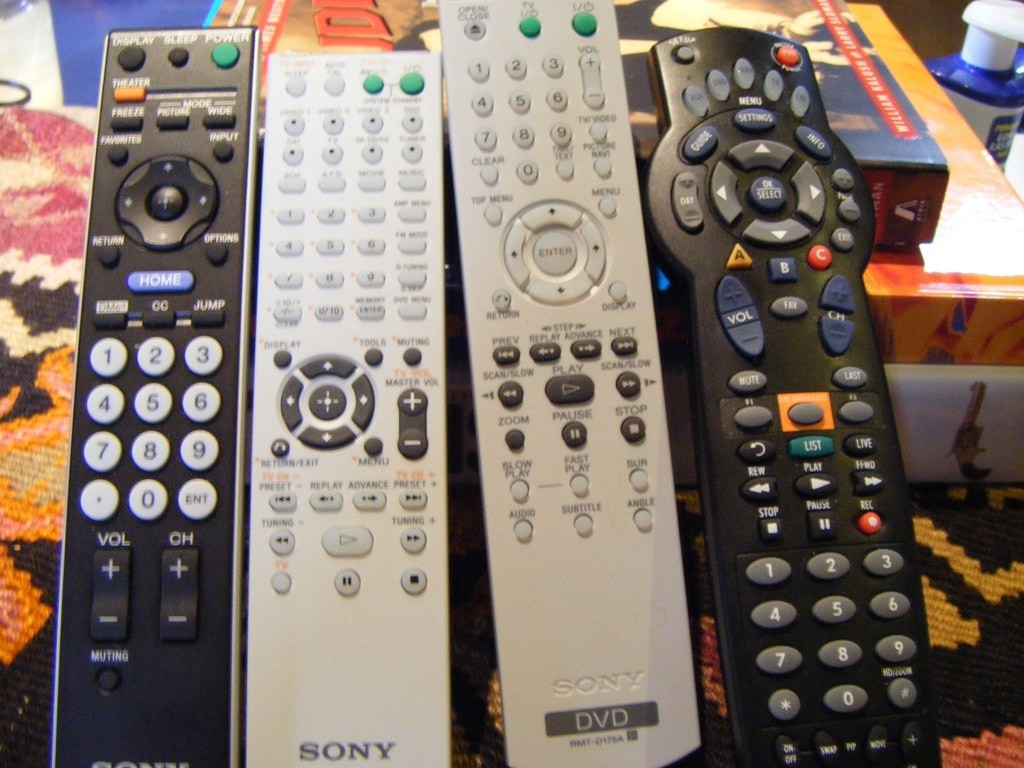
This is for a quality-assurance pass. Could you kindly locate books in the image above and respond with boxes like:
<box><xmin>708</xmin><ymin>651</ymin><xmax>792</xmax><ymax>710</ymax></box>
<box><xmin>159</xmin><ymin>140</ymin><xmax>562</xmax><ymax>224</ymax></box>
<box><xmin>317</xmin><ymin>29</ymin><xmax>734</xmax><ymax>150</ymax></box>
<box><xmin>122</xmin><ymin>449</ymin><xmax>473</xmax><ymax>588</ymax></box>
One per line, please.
<box><xmin>199</xmin><ymin>0</ymin><xmax>1024</xmax><ymax>488</ymax></box>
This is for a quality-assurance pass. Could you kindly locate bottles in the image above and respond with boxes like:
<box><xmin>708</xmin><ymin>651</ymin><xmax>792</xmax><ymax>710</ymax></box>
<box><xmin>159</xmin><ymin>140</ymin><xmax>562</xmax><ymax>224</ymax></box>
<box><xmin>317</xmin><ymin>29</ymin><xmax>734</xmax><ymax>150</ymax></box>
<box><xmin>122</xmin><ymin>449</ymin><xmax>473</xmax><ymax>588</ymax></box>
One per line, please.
<box><xmin>924</xmin><ymin>0</ymin><xmax>1024</xmax><ymax>171</ymax></box>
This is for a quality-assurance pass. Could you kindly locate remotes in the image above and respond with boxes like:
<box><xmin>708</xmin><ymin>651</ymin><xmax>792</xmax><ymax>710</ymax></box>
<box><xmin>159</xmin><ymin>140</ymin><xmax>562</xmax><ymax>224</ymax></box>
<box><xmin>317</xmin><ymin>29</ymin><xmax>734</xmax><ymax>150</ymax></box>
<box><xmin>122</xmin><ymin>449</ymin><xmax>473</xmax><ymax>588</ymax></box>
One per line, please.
<box><xmin>48</xmin><ymin>0</ymin><xmax>944</xmax><ymax>768</ymax></box>
